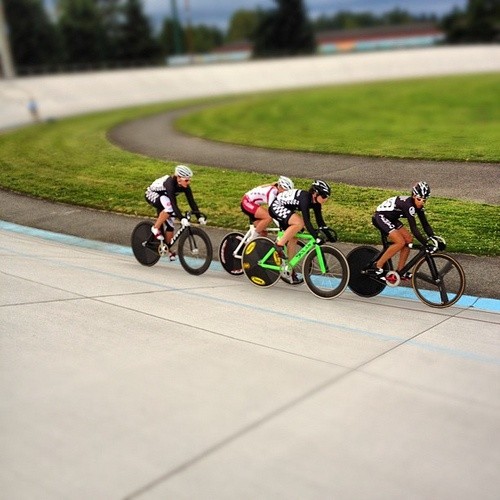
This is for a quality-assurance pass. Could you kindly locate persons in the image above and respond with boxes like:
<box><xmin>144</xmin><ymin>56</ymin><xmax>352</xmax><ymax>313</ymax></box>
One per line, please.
<box><xmin>240</xmin><ymin>175</ymin><xmax>295</xmax><ymax>243</ymax></box>
<box><xmin>268</xmin><ymin>179</ymin><xmax>336</xmax><ymax>283</ymax></box>
<box><xmin>145</xmin><ymin>165</ymin><xmax>207</xmax><ymax>261</ymax></box>
<box><xmin>375</xmin><ymin>181</ymin><xmax>446</xmax><ymax>283</ymax></box>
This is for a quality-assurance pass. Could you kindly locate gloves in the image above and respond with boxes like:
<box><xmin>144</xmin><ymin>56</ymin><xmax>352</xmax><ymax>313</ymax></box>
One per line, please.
<box><xmin>427</xmin><ymin>244</ymin><xmax>439</xmax><ymax>253</ymax></box>
<box><xmin>198</xmin><ymin>217</ymin><xmax>207</xmax><ymax>225</ymax></box>
<box><xmin>316</xmin><ymin>238</ymin><xmax>324</xmax><ymax>245</ymax></box>
<box><xmin>181</xmin><ymin>218</ymin><xmax>190</xmax><ymax>226</ymax></box>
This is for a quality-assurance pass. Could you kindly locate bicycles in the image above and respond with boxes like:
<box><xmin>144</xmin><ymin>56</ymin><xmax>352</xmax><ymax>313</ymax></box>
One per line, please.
<box><xmin>345</xmin><ymin>216</ymin><xmax>465</xmax><ymax>309</ymax></box>
<box><xmin>131</xmin><ymin>211</ymin><xmax>213</xmax><ymax>275</ymax></box>
<box><xmin>218</xmin><ymin>217</ymin><xmax>350</xmax><ymax>300</ymax></box>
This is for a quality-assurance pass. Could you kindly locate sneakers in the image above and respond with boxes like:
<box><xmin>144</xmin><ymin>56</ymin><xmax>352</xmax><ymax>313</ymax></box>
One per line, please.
<box><xmin>151</xmin><ymin>226</ymin><xmax>165</xmax><ymax>241</ymax></box>
<box><xmin>169</xmin><ymin>251</ymin><xmax>175</xmax><ymax>261</ymax></box>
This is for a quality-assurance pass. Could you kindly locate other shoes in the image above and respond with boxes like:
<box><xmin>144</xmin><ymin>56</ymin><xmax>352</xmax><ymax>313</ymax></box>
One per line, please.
<box><xmin>403</xmin><ymin>273</ymin><xmax>412</xmax><ymax>278</ymax></box>
<box><xmin>292</xmin><ymin>271</ymin><xmax>299</xmax><ymax>282</ymax></box>
<box><xmin>274</xmin><ymin>243</ymin><xmax>287</xmax><ymax>259</ymax></box>
<box><xmin>372</xmin><ymin>262</ymin><xmax>385</xmax><ymax>277</ymax></box>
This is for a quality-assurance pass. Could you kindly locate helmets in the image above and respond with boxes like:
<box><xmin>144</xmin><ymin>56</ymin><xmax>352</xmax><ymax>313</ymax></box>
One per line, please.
<box><xmin>412</xmin><ymin>182</ymin><xmax>431</xmax><ymax>198</ymax></box>
<box><xmin>175</xmin><ymin>165</ymin><xmax>192</xmax><ymax>177</ymax></box>
<box><xmin>312</xmin><ymin>180</ymin><xmax>330</xmax><ymax>196</ymax></box>
<box><xmin>279</xmin><ymin>175</ymin><xmax>294</xmax><ymax>190</ymax></box>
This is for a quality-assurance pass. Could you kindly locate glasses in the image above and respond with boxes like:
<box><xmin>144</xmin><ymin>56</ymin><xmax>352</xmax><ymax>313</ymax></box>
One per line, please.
<box><xmin>181</xmin><ymin>178</ymin><xmax>191</xmax><ymax>182</ymax></box>
<box><xmin>318</xmin><ymin>193</ymin><xmax>328</xmax><ymax>199</ymax></box>
<box><xmin>416</xmin><ymin>198</ymin><xmax>426</xmax><ymax>203</ymax></box>
